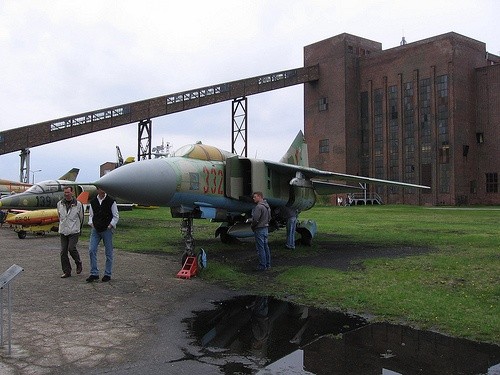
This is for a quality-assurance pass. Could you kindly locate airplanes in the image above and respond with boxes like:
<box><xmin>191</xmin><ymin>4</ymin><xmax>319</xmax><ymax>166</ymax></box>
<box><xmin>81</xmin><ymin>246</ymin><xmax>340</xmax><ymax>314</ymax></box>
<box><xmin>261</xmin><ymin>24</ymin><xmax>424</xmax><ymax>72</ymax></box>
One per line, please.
<box><xmin>45</xmin><ymin>129</ymin><xmax>431</xmax><ymax>279</ymax></box>
<box><xmin>6</xmin><ymin>191</ymin><xmax>88</xmax><ymax>239</ymax></box>
<box><xmin>0</xmin><ymin>168</ymin><xmax>139</xmax><ymax>239</ymax></box>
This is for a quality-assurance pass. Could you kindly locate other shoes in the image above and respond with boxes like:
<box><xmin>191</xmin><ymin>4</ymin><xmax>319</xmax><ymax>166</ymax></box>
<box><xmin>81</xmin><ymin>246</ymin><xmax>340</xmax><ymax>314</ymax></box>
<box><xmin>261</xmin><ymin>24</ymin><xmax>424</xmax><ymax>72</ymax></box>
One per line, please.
<box><xmin>77</xmin><ymin>265</ymin><xmax>82</xmax><ymax>274</ymax></box>
<box><xmin>60</xmin><ymin>273</ymin><xmax>71</xmax><ymax>278</ymax></box>
<box><xmin>86</xmin><ymin>275</ymin><xmax>99</xmax><ymax>282</ymax></box>
<box><xmin>102</xmin><ymin>275</ymin><xmax>111</xmax><ymax>282</ymax></box>
<box><xmin>255</xmin><ymin>264</ymin><xmax>266</xmax><ymax>271</ymax></box>
<box><xmin>266</xmin><ymin>264</ymin><xmax>271</xmax><ymax>270</ymax></box>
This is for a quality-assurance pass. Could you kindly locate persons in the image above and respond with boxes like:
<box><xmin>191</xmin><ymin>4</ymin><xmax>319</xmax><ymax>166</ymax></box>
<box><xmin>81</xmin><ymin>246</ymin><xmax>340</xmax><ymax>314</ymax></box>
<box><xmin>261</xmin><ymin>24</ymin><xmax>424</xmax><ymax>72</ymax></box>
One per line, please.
<box><xmin>338</xmin><ymin>193</ymin><xmax>354</xmax><ymax>207</ymax></box>
<box><xmin>251</xmin><ymin>192</ymin><xmax>271</xmax><ymax>273</ymax></box>
<box><xmin>86</xmin><ymin>185</ymin><xmax>119</xmax><ymax>282</ymax></box>
<box><xmin>57</xmin><ymin>187</ymin><xmax>84</xmax><ymax>278</ymax></box>
